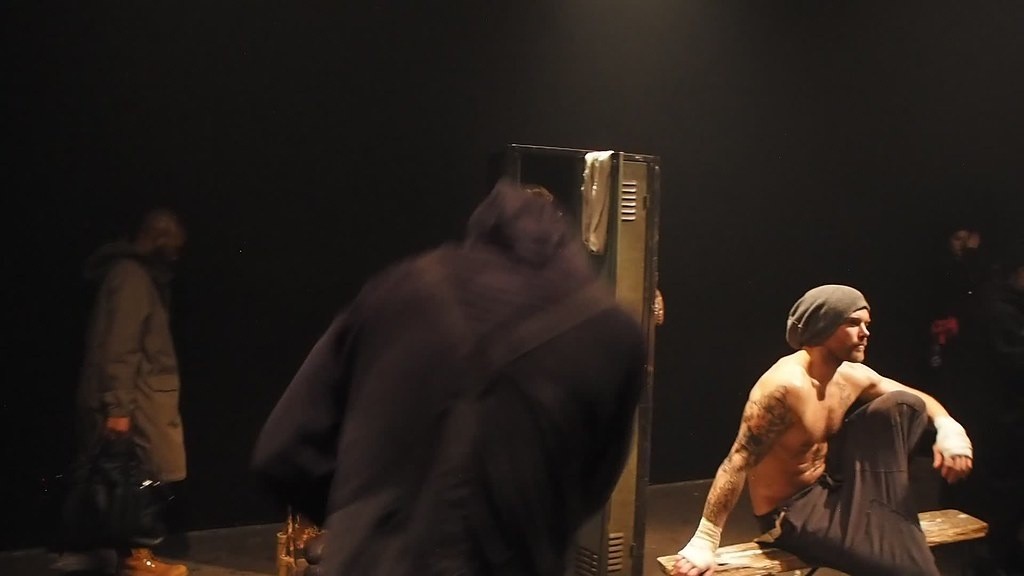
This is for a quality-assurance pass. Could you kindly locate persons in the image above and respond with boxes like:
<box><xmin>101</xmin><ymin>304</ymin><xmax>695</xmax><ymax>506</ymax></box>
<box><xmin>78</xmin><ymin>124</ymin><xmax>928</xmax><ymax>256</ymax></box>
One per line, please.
<box><xmin>252</xmin><ymin>179</ymin><xmax>649</xmax><ymax>576</ymax></box>
<box><xmin>77</xmin><ymin>206</ymin><xmax>189</xmax><ymax>576</ymax></box>
<box><xmin>668</xmin><ymin>285</ymin><xmax>975</xmax><ymax>576</ymax></box>
<box><xmin>920</xmin><ymin>214</ymin><xmax>1024</xmax><ymax>409</ymax></box>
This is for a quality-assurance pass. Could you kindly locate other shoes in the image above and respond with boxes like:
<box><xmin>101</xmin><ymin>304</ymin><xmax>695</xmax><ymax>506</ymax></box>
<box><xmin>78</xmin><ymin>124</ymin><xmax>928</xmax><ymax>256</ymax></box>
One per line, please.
<box><xmin>117</xmin><ymin>548</ymin><xmax>188</xmax><ymax>576</ymax></box>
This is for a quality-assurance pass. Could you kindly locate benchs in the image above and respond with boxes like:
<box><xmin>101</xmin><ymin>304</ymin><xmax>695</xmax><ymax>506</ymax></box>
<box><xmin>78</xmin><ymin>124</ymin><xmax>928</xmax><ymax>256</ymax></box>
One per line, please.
<box><xmin>656</xmin><ymin>491</ymin><xmax>989</xmax><ymax>576</ymax></box>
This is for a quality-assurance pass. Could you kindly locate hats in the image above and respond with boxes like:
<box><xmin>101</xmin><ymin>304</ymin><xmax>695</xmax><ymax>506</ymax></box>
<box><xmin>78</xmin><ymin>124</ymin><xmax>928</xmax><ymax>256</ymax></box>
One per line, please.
<box><xmin>786</xmin><ymin>283</ymin><xmax>871</xmax><ymax>351</ymax></box>
